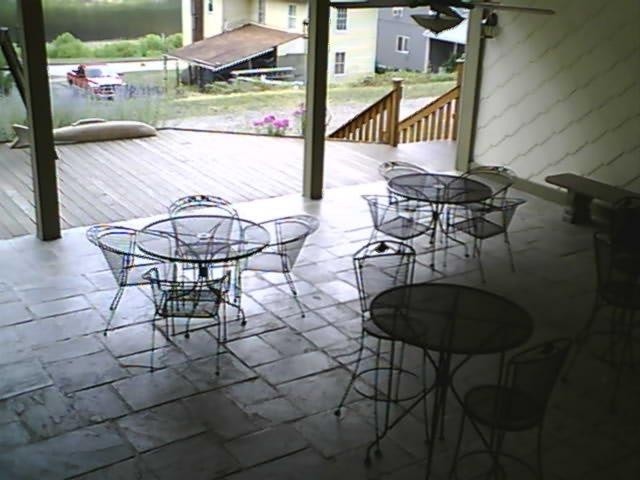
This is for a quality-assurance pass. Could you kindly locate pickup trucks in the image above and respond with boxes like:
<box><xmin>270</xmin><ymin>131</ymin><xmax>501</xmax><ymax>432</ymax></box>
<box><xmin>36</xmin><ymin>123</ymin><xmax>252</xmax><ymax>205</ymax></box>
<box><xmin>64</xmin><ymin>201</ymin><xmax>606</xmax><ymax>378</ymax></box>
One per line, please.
<box><xmin>67</xmin><ymin>62</ymin><xmax>130</xmax><ymax>100</ymax></box>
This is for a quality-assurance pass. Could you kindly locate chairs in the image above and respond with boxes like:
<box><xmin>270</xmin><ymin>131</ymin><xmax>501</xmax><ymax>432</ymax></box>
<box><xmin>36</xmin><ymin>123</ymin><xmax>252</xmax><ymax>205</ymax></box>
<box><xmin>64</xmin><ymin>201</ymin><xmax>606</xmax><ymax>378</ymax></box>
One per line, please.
<box><xmin>446</xmin><ymin>337</ymin><xmax>572</xmax><ymax>479</ymax></box>
<box><xmin>561</xmin><ymin>195</ymin><xmax>640</xmax><ymax>415</ymax></box>
<box><xmin>335</xmin><ymin>240</ymin><xmax>445</xmax><ymax>458</ymax></box>
<box><xmin>359</xmin><ymin>161</ymin><xmax>525</xmax><ymax>284</ymax></box>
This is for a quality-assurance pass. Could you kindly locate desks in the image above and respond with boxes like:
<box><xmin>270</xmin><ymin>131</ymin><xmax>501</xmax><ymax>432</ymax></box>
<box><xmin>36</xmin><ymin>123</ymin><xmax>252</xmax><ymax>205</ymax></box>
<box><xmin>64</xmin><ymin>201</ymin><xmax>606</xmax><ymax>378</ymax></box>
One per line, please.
<box><xmin>364</xmin><ymin>284</ymin><xmax>532</xmax><ymax>480</ymax></box>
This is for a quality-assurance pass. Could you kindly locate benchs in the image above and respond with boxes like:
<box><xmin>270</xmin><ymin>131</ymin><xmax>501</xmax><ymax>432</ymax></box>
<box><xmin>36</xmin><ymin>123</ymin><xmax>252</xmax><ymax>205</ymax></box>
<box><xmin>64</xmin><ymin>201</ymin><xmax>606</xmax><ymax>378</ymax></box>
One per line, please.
<box><xmin>545</xmin><ymin>173</ymin><xmax>640</xmax><ymax>224</ymax></box>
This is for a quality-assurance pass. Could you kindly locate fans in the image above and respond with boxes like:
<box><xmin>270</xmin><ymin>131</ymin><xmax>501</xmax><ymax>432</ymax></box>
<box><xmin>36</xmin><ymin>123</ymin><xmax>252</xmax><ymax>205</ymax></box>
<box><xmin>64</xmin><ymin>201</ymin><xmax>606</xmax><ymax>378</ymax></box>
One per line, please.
<box><xmin>330</xmin><ymin>1</ymin><xmax>555</xmax><ymax>37</ymax></box>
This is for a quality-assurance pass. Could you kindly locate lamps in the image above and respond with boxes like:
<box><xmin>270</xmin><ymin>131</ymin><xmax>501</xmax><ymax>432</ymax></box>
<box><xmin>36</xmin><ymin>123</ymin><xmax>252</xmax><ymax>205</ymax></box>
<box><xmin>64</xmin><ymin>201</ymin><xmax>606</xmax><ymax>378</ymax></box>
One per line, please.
<box><xmin>482</xmin><ymin>6</ymin><xmax>499</xmax><ymax>39</ymax></box>
<box><xmin>410</xmin><ymin>11</ymin><xmax>465</xmax><ymax>36</ymax></box>
